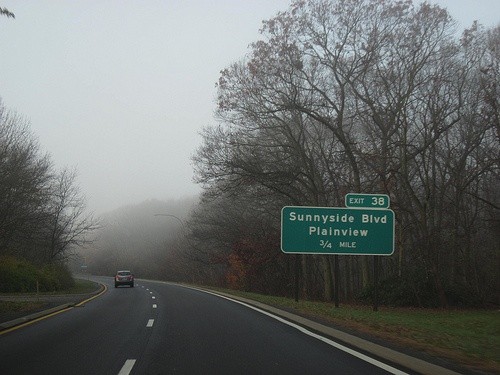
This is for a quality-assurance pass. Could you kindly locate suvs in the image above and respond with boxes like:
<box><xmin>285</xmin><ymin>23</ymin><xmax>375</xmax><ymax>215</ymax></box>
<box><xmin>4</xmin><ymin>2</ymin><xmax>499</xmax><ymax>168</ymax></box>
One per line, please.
<box><xmin>114</xmin><ymin>270</ymin><xmax>134</xmax><ymax>288</ymax></box>
